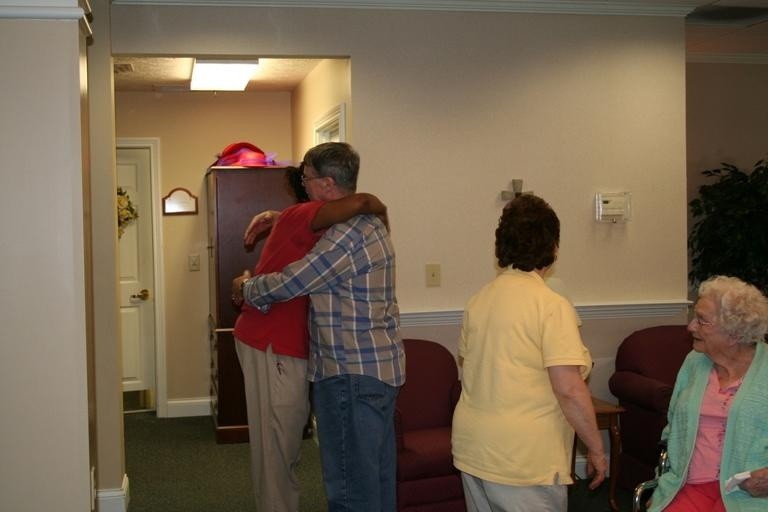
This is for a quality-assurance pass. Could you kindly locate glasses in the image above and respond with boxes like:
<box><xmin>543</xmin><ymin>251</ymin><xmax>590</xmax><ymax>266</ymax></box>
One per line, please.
<box><xmin>301</xmin><ymin>174</ymin><xmax>327</xmax><ymax>184</ymax></box>
<box><xmin>697</xmin><ymin>317</ymin><xmax>714</xmax><ymax>328</ymax></box>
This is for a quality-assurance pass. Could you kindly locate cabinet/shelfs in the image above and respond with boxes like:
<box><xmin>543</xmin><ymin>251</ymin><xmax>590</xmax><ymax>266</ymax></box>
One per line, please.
<box><xmin>204</xmin><ymin>164</ymin><xmax>309</xmax><ymax>445</ymax></box>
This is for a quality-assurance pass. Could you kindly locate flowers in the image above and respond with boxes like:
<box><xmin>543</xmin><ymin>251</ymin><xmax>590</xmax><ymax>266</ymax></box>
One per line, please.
<box><xmin>116</xmin><ymin>186</ymin><xmax>141</xmax><ymax>227</ymax></box>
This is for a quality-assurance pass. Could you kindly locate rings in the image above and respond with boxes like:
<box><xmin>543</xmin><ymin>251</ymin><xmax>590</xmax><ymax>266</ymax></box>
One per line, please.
<box><xmin>232</xmin><ymin>297</ymin><xmax>235</xmax><ymax>300</ymax></box>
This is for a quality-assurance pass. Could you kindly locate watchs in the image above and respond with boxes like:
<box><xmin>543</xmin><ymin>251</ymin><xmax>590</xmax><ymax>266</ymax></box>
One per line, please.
<box><xmin>240</xmin><ymin>279</ymin><xmax>248</xmax><ymax>298</ymax></box>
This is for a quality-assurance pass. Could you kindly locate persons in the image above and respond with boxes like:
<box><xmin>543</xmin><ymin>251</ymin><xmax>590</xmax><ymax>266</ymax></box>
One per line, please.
<box><xmin>647</xmin><ymin>276</ymin><xmax>768</xmax><ymax>512</ymax></box>
<box><xmin>450</xmin><ymin>192</ymin><xmax>608</xmax><ymax>512</ymax></box>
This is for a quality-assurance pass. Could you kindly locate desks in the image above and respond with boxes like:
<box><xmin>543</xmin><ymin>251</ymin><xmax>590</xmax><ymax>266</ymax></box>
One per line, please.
<box><xmin>569</xmin><ymin>397</ymin><xmax>627</xmax><ymax>512</ymax></box>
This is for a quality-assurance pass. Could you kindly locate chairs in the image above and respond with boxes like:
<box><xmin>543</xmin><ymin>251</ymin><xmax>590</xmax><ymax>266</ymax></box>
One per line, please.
<box><xmin>394</xmin><ymin>338</ymin><xmax>467</xmax><ymax>511</ymax></box>
<box><xmin>609</xmin><ymin>325</ymin><xmax>695</xmax><ymax>497</ymax></box>
